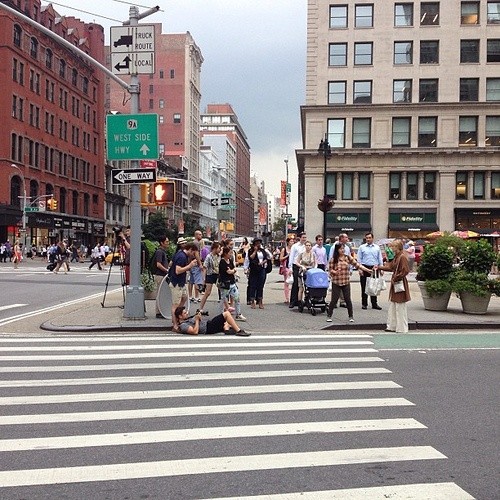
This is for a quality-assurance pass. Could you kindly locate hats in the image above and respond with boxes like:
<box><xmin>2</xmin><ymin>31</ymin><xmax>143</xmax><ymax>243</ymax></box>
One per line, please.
<box><xmin>176</xmin><ymin>237</ymin><xmax>187</xmax><ymax>244</ymax></box>
<box><xmin>252</xmin><ymin>237</ymin><xmax>263</xmax><ymax>244</ymax></box>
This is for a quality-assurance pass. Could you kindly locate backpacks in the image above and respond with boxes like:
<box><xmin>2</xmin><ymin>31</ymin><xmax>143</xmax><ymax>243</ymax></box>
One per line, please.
<box><xmin>148</xmin><ymin>248</ymin><xmax>159</xmax><ymax>274</ymax></box>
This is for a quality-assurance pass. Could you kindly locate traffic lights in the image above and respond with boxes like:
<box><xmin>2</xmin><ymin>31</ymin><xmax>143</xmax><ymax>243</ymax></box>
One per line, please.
<box><xmin>54</xmin><ymin>201</ymin><xmax>58</xmax><ymax>210</ymax></box>
<box><xmin>153</xmin><ymin>181</ymin><xmax>176</xmax><ymax>204</ymax></box>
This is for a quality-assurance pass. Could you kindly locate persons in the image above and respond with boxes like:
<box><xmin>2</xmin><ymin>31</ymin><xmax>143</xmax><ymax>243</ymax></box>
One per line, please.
<box><xmin>31</xmin><ymin>240</ymin><xmax>88</xmax><ymax>274</ymax></box>
<box><xmin>327</xmin><ymin>242</ymin><xmax>373</xmax><ymax>322</ymax></box>
<box><xmin>175</xmin><ymin>306</ymin><xmax>251</xmax><ymax>336</ymax></box>
<box><xmin>0</xmin><ymin>239</ymin><xmax>22</xmax><ymax>269</ymax></box>
<box><xmin>273</xmin><ymin>233</ymin><xmax>352</xmax><ymax>308</ymax></box>
<box><xmin>357</xmin><ymin>233</ymin><xmax>383</xmax><ymax>309</ymax></box>
<box><xmin>89</xmin><ymin>243</ymin><xmax>109</xmax><ymax>270</ymax></box>
<box><xmin>119</xmin><ymin>225</ymin><xmax>130</xmax><ymax>285</ymax></box>
<box><xmin>155</xmin><ymin>236</ymin><xmax>170</xmax><ymax>318</ymax></box>
<box><xmin>166</xmin><ymin>243</ymin><xmax>198</xmax><ymax>332</ymax></box>
<box><xmin>174</xmin><ymin>230</ymin><xmax>272</xmax><ymax>320</ymax></box>
<box><xmin>374</xmin><ymin>240</ymin><xmax>411</xmax><ymax>333</ymax></box>
<box><xmin>386</xmin><ymin>239</ymin><xmax>415</xmax><ymax>273</ymax></box>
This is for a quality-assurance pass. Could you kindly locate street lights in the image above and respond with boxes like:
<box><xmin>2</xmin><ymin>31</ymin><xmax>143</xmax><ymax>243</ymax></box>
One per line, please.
<box><xmin>10</xmin><ymin>164</ymin><xmax>27</xmax><ymax>257</ymax></box>
<box><xmin>317</xmin><ymin>133</ymin><xmax>333</xmax><ymax>243</ymax></box>
<box><xmin>284</xmin><ymin>156</ymin><xmax>290</xmax><ymax>241</ymax></box>
<box><xmin>245</xmin><ymin>197</ymin><xmax>269</xmax><ymax>233</ymax></box>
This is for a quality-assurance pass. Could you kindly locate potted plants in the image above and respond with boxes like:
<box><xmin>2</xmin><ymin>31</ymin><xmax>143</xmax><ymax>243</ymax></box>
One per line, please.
<box><xmin>417</xmin><ymin>278</ymin><xmax>453</xmax><ymax>311</ymax></box>
<box><xmin>452</xmin><ymin>268</ymin><xmax>493</xmax><ymax>315</ymax></box>
<box><xmin>139</xmin><ymin>270</ymin><xmax>158</xmax><ymax>301</ymax></box>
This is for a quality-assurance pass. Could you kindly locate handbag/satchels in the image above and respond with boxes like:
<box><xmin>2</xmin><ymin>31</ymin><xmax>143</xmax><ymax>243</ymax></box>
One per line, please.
<box><xmin>364</xmin><ymin>269</ymin><xmax>381</xmax><ymax>297</ymax></box>
<box><xmin>278</xmin><ymin>248</ymin><xmax>288</xmax><ymax>275</ymax></box>
<box><xmin>377</xmin><ymin>270</ymin><xmax>387</xmax><ymax>291</ymax></box>
<box><xmin>393</xmin><ymin>278</ymin><xmax>405</xmax><ymax>293</ymax></box>
<box><xmin>13</xmin><ymin>256</ymin><xmax>18</xmax><ymax>264</ymax></box>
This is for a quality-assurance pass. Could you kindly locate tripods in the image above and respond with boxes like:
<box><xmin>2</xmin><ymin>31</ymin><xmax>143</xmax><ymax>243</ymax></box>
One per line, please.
<box><xmin>101</xmin><ymin>233</ymin><xmax>125</xmax><ymax>308</ymax></box>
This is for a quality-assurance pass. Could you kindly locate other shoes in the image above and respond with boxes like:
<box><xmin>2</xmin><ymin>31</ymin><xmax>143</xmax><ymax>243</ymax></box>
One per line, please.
<box><xmin>196</xmin><ymin>298</ymin><xmax>202</xmax><ymax>302</ymax></box>
<box><xmin>362</xmin><ymin>304</ymin><xmax>368</xmax><ymax>309</ymax></box>
<box><xmin>189</xmin><ymin>296</ymin><xmax>195</xmax><ymax>302</ymax></box>
<box><xmin>223</xmin><ymin>305</ymin><xmax>235</xmax><ymax>312</ymax></box>
<box><xmin>372</xmin><ymin>305</ymin><xmax>382</xmax><ymax>310</ymax></box>
<box><xmin>349</xmin><ymin>316</ymin><xmax>355</xmax><ymax>322</ymax></box>
<box><xmin>251</xmin><ymin>302</ymin><xmax>256</xmax><ymax>309</ymax></box>
<box><xmin>258</xmin><ymin>303</ymin><xmax>264</xmax><ymax>309</ymax></box>
<box><xmin>224</xmin><ymin>327</ymin><xmax>235</xmax><ymax>334</ymax></box>
<box><xmin>327</xmin><ymin>316</ymin><xmax>333</xmax><ymax>322</ymax></box>
<box><xmin>235</xmin><ymin>329</ymin><xmax>252</xmax><ymax>336</ymax></box>
<box><xmin>235</xmin><ymin>315</ymin><xmax>246</xmax><ymax>321</ymax></box>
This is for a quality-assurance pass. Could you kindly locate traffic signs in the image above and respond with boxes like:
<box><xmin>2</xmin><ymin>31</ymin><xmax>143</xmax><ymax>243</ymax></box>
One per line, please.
<box><xmin>106</xmin><ymin>113</ymin><xmax>159</xmax><ymax>161</ymax></box>
<box><xmin>210</xmin><ymin>197</ymin><xmax>229</xmax><ymax>207</ymax></box>
<box><xmin>110</xmin><ymin>168</ymin><xmax>157</xmax><ymax>186</ymax></box>
<box><xmin>111</xmin><ymin>51</ymin><xmax>155</xmax><ymax>75</ymax></box>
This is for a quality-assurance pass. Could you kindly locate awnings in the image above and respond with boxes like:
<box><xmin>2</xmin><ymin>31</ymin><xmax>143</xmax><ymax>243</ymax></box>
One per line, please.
<box><xmin>327</xmin><ymin>223</ymin><xmax>370</xmax><ymax>231</ymax></box>
<box><xmin>390</xmin><ymin>224</ymin><xmax>440</xmax><ymax>232</ymax></box>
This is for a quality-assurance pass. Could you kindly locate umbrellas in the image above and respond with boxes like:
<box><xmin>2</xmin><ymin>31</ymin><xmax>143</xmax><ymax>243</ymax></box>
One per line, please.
<box><xmin>427</xmin><ymin>230</ymin><xmax>480</xmax><ymax>239</ymax></box>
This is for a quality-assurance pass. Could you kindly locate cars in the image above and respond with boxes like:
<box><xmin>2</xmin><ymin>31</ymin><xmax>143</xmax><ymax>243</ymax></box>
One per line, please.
<box><xmin>414</xmin><ymin>244</ymin><xmax>427</xmax><ymax>267</ymax></box>
<box><xmin>91</xmin><ymin>248</ymin><xmax>113</xmax><ymax>262</ymax></box>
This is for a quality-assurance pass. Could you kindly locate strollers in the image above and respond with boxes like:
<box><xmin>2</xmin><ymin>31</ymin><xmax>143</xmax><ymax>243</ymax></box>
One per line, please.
<box><xmin>297</xmin><ymin>266</ymin><xmax>331</xmax><ymax>316</ymax></box>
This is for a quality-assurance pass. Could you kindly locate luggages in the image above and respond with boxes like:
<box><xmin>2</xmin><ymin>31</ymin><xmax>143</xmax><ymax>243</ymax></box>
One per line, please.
<box><xmin>47</xmin><ymin>263</ymin><xmax>57</xmax><ymax>271</ymax></box>
